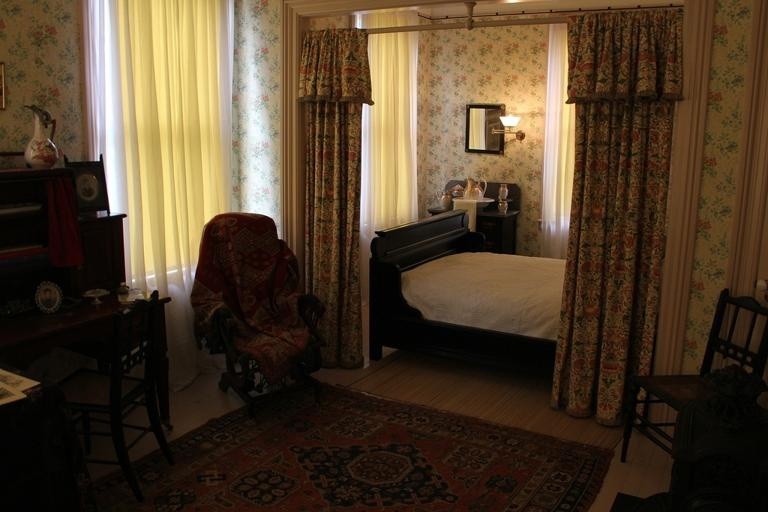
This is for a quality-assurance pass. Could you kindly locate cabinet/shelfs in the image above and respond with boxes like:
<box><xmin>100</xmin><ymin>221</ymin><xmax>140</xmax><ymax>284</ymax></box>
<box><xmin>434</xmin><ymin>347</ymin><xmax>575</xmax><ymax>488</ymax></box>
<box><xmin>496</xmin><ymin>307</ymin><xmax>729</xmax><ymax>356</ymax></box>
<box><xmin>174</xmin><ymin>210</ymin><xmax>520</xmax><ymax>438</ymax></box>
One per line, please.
<box><xmin>0</xmin><ymin>166</ymin><xmax>173</xmax><ymax>432</ymax></box>
<box><xmin>427</xmin><ymin>180</ymin><xmax>521</xmax><ymax>256</ymax></box>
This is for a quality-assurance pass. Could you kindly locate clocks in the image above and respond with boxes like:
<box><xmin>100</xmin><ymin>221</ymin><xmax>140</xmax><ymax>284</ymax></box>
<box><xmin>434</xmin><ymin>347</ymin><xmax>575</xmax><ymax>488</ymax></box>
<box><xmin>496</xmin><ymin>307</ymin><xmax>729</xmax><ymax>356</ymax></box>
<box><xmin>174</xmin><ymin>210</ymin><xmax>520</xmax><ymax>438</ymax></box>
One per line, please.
<box><xmin>64</xmin><ymin>153</ymin><xmax>110</xmax><ymax>217</ymax></box>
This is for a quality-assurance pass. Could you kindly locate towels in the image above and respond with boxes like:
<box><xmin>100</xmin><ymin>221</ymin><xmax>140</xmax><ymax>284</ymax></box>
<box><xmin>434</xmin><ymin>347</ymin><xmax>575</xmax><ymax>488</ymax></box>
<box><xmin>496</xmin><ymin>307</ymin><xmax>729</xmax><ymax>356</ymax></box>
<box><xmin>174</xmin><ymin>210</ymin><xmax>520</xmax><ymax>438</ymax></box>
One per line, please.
<box><xmin>453</xmin><ymin>198</ymin><xmax>477</xmax><ymax>233</ymax></box>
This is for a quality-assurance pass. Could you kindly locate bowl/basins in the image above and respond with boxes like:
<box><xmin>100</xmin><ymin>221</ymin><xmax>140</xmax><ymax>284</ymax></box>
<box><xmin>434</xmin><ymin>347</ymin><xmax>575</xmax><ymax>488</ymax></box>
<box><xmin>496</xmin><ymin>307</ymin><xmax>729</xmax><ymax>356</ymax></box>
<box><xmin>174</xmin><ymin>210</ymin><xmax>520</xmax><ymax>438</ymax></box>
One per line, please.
<box><xmin>452</xmin><ymin>197</ymin><xmax>495</xmax><ymax>208</ymax></box>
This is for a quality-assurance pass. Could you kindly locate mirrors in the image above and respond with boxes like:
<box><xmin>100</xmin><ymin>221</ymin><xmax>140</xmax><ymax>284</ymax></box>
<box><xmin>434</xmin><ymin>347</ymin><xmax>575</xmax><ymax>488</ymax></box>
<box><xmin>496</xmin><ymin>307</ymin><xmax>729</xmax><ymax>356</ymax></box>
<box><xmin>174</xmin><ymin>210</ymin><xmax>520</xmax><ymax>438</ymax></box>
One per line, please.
<box><xmin>465</xmin><ymin>104</ymin><xmax>504</xmax><ymax>156</ymax></box>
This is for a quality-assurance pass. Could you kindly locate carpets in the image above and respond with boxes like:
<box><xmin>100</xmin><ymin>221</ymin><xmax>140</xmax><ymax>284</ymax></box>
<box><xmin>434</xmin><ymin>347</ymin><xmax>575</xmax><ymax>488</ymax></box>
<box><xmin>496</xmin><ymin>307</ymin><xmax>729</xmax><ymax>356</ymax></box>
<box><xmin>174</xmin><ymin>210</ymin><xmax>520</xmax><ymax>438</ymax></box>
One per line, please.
<box><xmin>2</xmin><ymin>376</ymin><xmax>616</xmax><ymax>512</ymax></box>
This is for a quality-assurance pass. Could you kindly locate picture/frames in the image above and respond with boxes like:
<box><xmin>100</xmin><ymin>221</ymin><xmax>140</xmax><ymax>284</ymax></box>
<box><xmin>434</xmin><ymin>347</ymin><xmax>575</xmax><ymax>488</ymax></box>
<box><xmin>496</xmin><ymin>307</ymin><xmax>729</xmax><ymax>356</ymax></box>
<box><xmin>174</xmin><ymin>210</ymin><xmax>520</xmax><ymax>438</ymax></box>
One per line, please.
<box><xmin>0</xmin><ymin>61</ymin><xmax>5</xmax><ymax>110</ymax></box>
<box><xmin>34</xmin><ymin>279</ymin><xmax>65</xmax><ymax>316</ymax></box>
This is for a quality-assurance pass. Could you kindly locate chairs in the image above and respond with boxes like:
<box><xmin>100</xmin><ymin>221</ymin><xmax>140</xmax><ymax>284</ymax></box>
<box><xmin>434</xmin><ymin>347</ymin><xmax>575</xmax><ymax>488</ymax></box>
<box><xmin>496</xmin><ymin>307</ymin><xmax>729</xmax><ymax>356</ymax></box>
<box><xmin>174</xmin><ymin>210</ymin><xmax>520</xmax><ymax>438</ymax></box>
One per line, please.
<box><xmin>48</xmin><ymin>292</ymin><xmax>175</xmax><ymax>505</ymax></box>
<box><xmin>190</xmin><ymin>211</ymin><xmax>329</xmax><ymax>421</ymax></box>
<box><xmin>620</xmin><ymin>287</ymin><xmax>767</xmax><ymax>462</ymax></box>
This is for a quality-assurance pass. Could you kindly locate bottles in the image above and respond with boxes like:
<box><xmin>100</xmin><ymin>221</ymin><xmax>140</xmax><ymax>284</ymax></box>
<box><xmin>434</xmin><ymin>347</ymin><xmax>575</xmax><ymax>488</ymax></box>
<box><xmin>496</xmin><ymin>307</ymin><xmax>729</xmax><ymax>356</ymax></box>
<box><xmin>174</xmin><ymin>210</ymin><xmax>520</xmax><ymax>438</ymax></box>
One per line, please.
<box><xmin>116</xmin><ymin>281</ymin><xmax>130</xmax><ymax>305</ymax></box>
<box><xmin>498</xmin><ymin>201</ymin><xmax>508</xmax><ymax>215</ymax></box>
<box><xmin>499</xmin><ymin>184</ymin><xmax>508</xmax><ymax>200</ymax></box>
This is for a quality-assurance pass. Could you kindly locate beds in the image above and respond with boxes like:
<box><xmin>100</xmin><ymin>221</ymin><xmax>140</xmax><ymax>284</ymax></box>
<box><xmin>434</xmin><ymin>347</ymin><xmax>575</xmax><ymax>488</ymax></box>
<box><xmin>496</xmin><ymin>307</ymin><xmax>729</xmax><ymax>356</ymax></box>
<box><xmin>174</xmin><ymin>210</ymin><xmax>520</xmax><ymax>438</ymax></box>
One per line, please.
<box><xmin>368</xmin><ymin>213</ymin><xmax>567</xmax><ymax>382</ymax></box>
